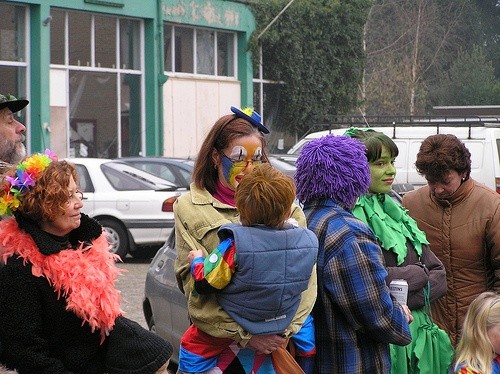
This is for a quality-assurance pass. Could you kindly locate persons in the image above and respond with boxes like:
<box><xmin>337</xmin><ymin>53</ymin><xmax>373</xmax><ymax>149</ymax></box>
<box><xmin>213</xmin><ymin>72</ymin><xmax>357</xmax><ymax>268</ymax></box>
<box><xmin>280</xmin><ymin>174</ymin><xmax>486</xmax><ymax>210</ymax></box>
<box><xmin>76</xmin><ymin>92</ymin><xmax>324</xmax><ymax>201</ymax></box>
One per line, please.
<box><xmin>402</xmin><ymin>134</ymin><xmax>500</xmax><ymax>374</ymax></box>
<box><xmin>172</xmin><ymin>105</ymin><xmax>318</xmax><ymax>374</ymax></box>
<box><xmin>107</xmin><ymin>314</ymin><xmax>174</xmax><ymax>374</ymax></box>
<box><xmin>0</xmin><ymin>92</ymin><xmax>30</xmax><ymax>177</ymax></box>
<box><xmin>344</xmin><ymin>128</ymin><xmax>455</xmax><ymax>374</ymax></box>
<box><xmin>176</xmin><ymin>163</ymin><xmax>320</xmax><ymax>374</ymax></box>
<box><xmin>0</xmin><ymin>148</ymin><xmax>122</xmax><ymax>374</ymax></box>
<box><xmin>293</xmin><ymin>134</ymin><xmax>414</xmax><ymax>374</ymax></box>
<box><xmin>452</xmin><ymin>290</ymin><xmax>500</xmax><ymax>374</ymax></box>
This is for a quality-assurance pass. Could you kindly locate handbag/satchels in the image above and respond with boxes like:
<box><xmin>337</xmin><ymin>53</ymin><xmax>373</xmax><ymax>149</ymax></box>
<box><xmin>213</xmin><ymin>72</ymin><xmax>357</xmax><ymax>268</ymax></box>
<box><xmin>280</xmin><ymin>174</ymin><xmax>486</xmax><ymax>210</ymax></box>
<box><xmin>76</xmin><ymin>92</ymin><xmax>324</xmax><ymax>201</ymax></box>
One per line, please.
<box><xmin>388</xmin><ymin>276</ymin><xmax>455</xmax><ymax>374</ymax></box>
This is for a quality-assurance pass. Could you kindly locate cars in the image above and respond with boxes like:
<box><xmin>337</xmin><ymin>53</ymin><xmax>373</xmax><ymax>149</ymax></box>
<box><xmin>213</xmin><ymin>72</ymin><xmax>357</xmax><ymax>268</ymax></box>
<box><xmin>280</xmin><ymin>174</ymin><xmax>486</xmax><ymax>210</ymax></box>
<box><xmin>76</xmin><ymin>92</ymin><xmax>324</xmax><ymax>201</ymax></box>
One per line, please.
<box><xmin>53</xmin><ymin>156</ymin><xmax>186</xmax><ymax>261</ymax></box>
<box><xmin>266</xmin><ymin>154</ymin><xmax>299</xmax><ymax>183</ymax></box>
<box><xmin>114</xmin><ymin>154</ymin><xmax>200</xmax><ymax>191</ymax></box>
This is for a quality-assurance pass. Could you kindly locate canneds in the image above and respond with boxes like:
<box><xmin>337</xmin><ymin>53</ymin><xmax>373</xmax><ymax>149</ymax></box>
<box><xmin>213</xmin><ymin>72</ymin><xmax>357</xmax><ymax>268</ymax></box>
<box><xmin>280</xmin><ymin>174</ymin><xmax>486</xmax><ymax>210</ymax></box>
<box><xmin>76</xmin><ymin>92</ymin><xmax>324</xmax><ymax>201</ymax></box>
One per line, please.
<box><xmin>390</xmin><ymin>278</ymin><xmax>408</xmax><ymax>308</ymax></box>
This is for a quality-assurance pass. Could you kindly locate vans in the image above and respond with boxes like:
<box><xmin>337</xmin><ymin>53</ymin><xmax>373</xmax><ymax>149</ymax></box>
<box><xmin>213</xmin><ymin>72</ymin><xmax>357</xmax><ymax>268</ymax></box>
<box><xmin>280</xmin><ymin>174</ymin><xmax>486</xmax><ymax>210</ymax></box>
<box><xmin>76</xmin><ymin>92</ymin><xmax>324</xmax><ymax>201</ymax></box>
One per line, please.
<box><xmin>284</xmin><ymin>115</ymin><xmax>500</xmax><ymax>197</ymax></box>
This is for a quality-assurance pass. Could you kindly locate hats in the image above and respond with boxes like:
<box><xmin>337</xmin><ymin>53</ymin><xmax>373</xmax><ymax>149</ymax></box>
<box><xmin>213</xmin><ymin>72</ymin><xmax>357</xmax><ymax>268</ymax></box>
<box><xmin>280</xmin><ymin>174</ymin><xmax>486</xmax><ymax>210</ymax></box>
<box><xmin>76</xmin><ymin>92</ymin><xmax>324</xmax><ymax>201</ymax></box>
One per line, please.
<box><xmin>231</xmin><ymin>106</ymin><xmax>271</xmax><ymax>134</ymax></box>
<box><xmin>0</xmin><ymin>94</ymin><xmax>29</xmax><ymax>115</ymax></box>
